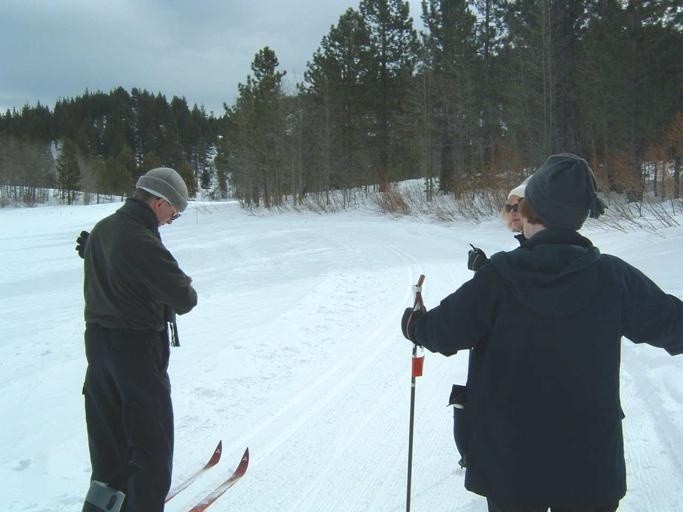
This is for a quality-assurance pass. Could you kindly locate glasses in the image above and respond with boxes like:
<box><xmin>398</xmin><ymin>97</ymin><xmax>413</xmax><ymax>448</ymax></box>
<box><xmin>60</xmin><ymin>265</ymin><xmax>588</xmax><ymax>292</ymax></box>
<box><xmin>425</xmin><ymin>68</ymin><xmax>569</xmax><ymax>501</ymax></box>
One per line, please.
<box><xmin>505</xmin><ymin>203</ymin><xmax>519</xmax><ymax>213</ymax></box>
<box><xmin>156</xmin><ymin>196</ymin><xmax>181</xmax><ymax>220</ymax></box>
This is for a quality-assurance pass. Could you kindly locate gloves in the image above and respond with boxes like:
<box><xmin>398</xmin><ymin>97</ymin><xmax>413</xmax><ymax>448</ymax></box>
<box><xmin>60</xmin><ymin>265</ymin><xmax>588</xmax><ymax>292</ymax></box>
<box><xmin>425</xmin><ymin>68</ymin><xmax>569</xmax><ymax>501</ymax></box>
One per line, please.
<box><xmin>75</xmin><ymin>231</ymin><xmax>89</xmax><ymax>258</ymax></box>
<box><xmin>400</xmin><ymin>307</ymin><xmax>427</xmax><ymax>348</ymax></box>
<box><xmin>466</xmin><ymin>249</ymin><xmax>488</xmax><ymax>271</ymax></box>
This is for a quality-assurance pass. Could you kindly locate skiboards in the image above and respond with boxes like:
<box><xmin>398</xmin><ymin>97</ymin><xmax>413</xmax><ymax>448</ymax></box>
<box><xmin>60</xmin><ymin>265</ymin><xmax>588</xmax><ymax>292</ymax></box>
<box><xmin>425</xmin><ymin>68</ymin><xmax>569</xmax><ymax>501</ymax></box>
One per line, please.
<box><xmin>164</xmin><ymin>440</ymin><xmax>249</xmax><ymax>512</ymax></box>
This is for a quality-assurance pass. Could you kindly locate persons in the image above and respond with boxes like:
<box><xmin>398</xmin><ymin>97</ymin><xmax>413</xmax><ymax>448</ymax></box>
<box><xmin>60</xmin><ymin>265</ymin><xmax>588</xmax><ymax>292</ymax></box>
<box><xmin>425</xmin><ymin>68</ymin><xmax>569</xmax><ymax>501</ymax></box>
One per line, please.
<box><xmin>399</xmin><ymin>152</ymin><xmax>681</xmax><ymax>512</ymax></box>
<box><xmin>465</xmin><ymin>173</ymin><xmax>535</xmax><ymax>279</ymax></box>
<box><xmin>78</xmin><ymin>162</ymin><xmax>199</xmax><ymax>512</ymax></box>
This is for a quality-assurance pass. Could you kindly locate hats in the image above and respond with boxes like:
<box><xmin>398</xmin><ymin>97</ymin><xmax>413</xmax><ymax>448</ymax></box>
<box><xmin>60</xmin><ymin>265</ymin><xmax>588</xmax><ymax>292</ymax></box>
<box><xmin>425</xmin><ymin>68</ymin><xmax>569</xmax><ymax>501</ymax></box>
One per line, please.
<box><xmin>507</xmin><ymin>150</ymin><xmax>606</xmax><ymax>231</ymax></box>
<box><xmin>136</xmin><ymin>168</ymin><xmax>189</xmax><ymax>214</ymax></box>
